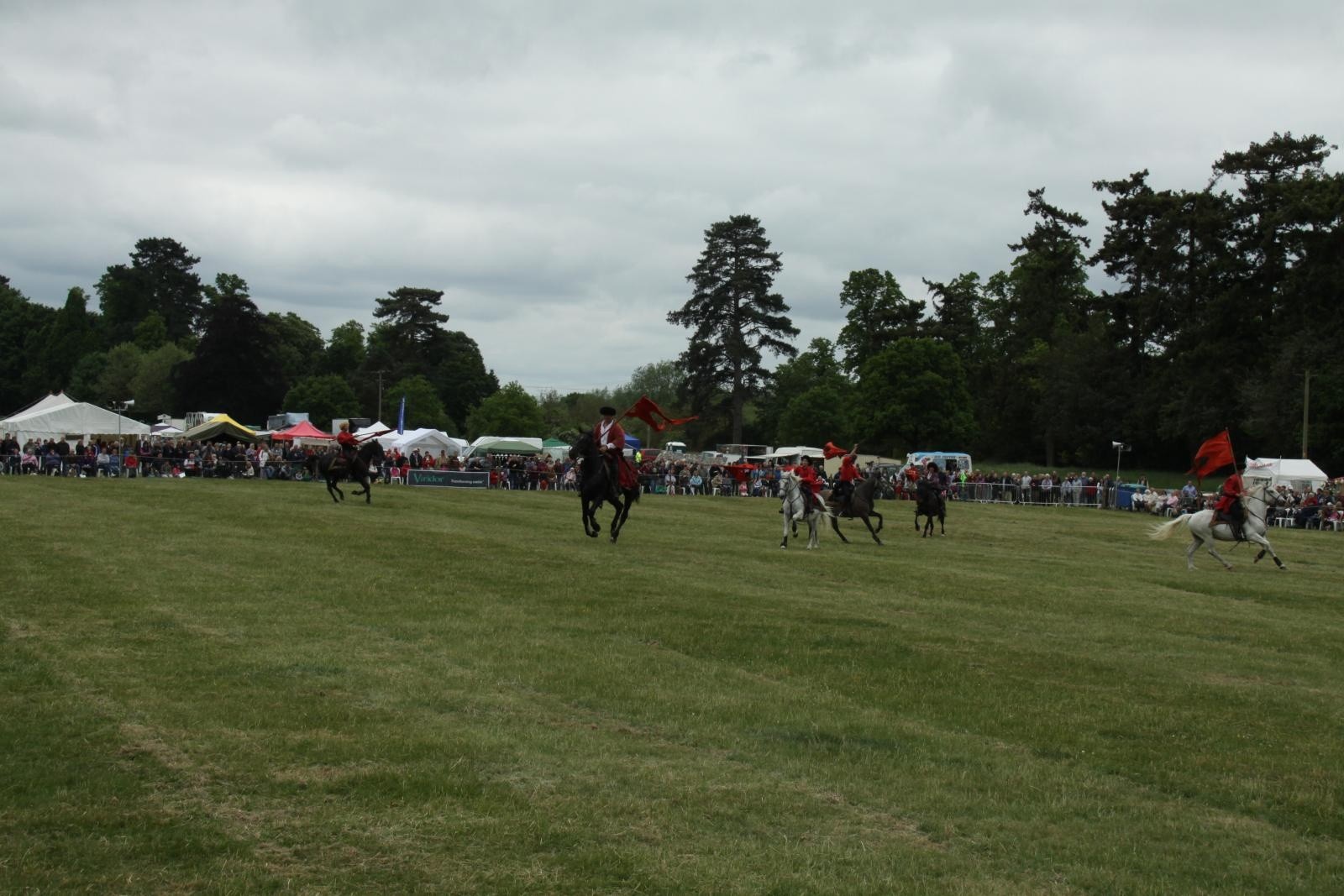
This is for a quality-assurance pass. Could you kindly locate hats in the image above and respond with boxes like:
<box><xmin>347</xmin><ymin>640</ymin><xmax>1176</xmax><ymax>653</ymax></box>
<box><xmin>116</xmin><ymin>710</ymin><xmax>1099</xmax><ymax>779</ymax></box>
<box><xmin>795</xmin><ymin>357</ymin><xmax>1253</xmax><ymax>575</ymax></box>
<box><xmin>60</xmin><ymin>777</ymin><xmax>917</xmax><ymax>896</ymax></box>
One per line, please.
<box><xmin>600</xmin><ymin>407</ymin><xmax>617</xmax><ymax>417</ymax></box>
<box><xmin>340</xmin><ymin>422</ymin><xmax>350</xmax><ymax>429</ymax></box>
<box><xmin>802</xmin><ymin>455</ymin><xmax>812</xmax><ymax>466</ymax></box>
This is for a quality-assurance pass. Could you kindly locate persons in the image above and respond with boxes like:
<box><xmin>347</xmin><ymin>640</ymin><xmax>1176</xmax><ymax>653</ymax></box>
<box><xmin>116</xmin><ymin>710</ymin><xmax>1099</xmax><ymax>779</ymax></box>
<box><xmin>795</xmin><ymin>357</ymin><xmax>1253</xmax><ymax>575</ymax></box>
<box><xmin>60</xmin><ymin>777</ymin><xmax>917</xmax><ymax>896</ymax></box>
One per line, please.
<box><xmin>856</xmin><ymin>456</ymin><xmax>1114</xmax><ymax>517</ymax></box>
<box><xmin>0</xmin><ymin>433</ymin><xmax>342</xmax><ymax>482</ymax></box>
<box><xmin>838</xmin><ymin>443</ymin><xmax>866</xmax><ymax>516</ymax></box>
<box><xmin>593</xmin><ymin>407</ymin><xmax>625</xmax><ymax>496</ymax></box>
<box><xmin>336</xmin><ymin>422</ymin><xmax>363</xmax><ymax>473</ymax></box>
<box><xmin>368</xmin><ymin>448</ymin><xmax>584</xmax><ymax>496</ymax></box>
<box><xmin>1265</xmin><ymin>478</ymin><xmax>1344</xmax><ymax>532</ymax></box>
<box><xmin>1215</xmin><ymin>463</ymin><xmax>1248</xmax><ymax>539</ymax></box>
<box><xmin>813</xmin><ymin>465</ymin><xmax>842</xmax><ymax>491</ymax></box>
<box><xmin>1114</xmin><ymin>474</ymin><xmax>1219</xmax><ymax>518</ymax></box>
<box><xmin>632</xmin><ymin>448</ymin><xmax>780</xmax><ymax>497</ymax></box>
<box><xmin>778</xmin><ymin>455</ymin><xmax>815</xmax><ymax>513</ymax></box>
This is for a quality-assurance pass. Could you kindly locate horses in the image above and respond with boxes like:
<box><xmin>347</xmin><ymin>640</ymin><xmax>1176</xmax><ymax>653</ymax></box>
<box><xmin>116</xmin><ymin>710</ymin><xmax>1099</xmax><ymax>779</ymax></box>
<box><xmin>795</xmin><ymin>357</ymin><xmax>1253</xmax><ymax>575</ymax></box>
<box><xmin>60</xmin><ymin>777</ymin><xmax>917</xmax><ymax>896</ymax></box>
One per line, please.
<box><xmin>912</xmin><ymin>479</ymin><xmax>950</xmax><ymax>538</ymax></box>
<box><xmin>569</xmin><ymin>425</ymin><xmax>651</xmax><ymax>544</ymax></box>
<box><xmin>306</xmin><ymin>438</ymin><xmax>387</xmax><ymax>504</ymax></box>
<box><xmin>1142</xmin><ymin>481</ymin><xmax>1287</xmax><ymax>572</ymax></box>
<box><xmin>777</xmin><ymin>468</ymin><xmax>838</xmax><ymax>550</ymax></box>
<box><xmin>829</xmin><ymin>471</ymin><xmax>885</xmax><ymax>546</ymax></box>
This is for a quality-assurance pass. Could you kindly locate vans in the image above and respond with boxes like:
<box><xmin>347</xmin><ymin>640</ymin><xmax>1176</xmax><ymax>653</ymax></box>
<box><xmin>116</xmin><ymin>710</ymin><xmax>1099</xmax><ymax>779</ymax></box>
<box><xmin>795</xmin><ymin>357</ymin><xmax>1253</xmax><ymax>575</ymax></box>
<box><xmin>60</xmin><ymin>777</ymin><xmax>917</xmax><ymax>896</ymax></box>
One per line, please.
<box><xmin>639</xmin><ymin>448</ymin><xmax>661</xmax><ymax>465</ymax></box>
<box><xmin>906</xmin><ymin>451</ymin><xmax>972</xmax><ymax>475</ymax></box>
<box><xmin>870</xmin><ymin>463</ymin><xmax>903</xmax><ymax>477</ymax></box>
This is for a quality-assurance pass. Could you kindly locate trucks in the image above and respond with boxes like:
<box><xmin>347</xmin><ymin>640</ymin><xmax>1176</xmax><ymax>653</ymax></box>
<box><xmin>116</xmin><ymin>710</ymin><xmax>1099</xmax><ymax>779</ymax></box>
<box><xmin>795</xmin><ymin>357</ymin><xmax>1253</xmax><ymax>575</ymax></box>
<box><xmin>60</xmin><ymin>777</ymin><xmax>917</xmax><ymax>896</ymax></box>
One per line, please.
<box><xmin>700</xmin><ymin>443</ymin><xmax>773</xmax><ymax>470</ymax></box>
<box><xmin>742</xmin><ymin>445</ymin><xmax>825</xmax><ymax>468</ymax></box>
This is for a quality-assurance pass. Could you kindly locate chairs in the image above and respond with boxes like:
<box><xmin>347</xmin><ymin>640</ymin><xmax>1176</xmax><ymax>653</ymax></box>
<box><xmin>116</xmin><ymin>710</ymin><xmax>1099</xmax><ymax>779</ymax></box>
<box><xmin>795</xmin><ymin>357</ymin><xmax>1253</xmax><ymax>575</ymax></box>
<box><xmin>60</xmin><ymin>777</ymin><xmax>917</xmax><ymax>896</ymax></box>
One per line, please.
<box><xmin>640</xmin><ymin>483</ymin><xmax>775</xmax><ymax>499</ymax></box>
<box><xmin>1131</xmin><ymin>493</ymin><xmax>1343</xmax><ymax>532</ymax></box>
<box><xmin>8</xmin><ymin>456</ymin><xmax>143</xmax><ymax>477</ymax></box>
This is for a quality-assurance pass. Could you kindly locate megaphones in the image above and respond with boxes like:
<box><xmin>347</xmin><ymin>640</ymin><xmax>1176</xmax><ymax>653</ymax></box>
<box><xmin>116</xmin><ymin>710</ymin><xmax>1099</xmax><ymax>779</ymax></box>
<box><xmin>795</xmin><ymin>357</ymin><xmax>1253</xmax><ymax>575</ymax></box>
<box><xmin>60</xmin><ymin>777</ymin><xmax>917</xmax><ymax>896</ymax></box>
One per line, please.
<box><xmin>123</xmin><ymin>399</ymin><xmax>134</xmax><ymax>406</ymax></box>
<box><xmin>1112</xmin><ymin>442</ymin><xmax>1132</xmax><ymax>451</ymax></box>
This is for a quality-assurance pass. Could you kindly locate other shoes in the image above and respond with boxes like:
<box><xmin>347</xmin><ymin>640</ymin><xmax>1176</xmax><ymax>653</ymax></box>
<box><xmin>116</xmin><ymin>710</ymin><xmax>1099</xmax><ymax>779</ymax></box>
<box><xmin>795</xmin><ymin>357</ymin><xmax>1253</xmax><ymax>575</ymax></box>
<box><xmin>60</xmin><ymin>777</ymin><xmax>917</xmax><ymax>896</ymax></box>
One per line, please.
<box><xmin>779</xmin><ymin>510</ymin><xmax>784</xmax><ymax>513</ymax></box>
<box><xmin>1237</xmin><ymin>533</ymin><xmax>1243</xmax><ymax>539</ymax></box>
<box><xmin>613</xmin><ymin>493</ymin><xmax>619</xmax><ymax>498</ymax></box>
<box><xmin>806</xmin><ymin>509</ymin><xmax>811</xmax><ymax>514</ymax></box>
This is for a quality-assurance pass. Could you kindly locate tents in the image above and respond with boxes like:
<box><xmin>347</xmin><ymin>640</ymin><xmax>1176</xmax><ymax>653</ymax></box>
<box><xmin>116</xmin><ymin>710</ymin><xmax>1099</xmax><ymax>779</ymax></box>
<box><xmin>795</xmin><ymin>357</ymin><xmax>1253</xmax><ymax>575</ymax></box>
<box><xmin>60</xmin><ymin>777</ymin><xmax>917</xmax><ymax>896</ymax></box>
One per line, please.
<box><xmin>0</xmin><ymin>393</ymin><xmax>573</xmax><ymax>460</ymax></box>
<box><xmin>1241</xmin><ymin>455</ymin><xmax>1329</xmax><ymax>494</ymax></box>
